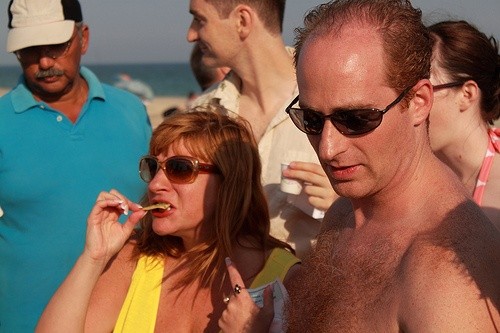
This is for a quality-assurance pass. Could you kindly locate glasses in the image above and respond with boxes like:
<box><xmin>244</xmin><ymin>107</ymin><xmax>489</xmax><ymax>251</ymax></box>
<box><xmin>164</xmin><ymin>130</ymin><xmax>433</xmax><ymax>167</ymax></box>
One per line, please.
<box><xmin>285</xmin><ymin>86</ymin><xmax>414</xmax><ymax>135</ymax></box>
<box><xmin>139</xmin><ymin>155</ymin><xmax>223</xmax><ymax>183</ymax></box>
<box><xmin>14</xmin><ymin>28</ymin><xmax>79</xmax><ymax>63</ymax></box>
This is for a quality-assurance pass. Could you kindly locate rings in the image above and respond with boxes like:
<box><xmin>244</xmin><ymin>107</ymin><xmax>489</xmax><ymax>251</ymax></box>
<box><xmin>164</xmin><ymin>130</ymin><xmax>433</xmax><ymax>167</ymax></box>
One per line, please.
<box><xmin>233</xmin><ymin>285</ymin><xmax>247</xmax><ymax>293</ymax></box>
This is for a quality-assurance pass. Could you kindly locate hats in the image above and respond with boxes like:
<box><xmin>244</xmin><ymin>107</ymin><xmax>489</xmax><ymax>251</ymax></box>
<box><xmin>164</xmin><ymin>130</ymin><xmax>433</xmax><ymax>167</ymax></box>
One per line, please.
<box><xmin>7</xmin><ymin>0</ymin><xmax>83</xmax><ymax>54</ymax></box>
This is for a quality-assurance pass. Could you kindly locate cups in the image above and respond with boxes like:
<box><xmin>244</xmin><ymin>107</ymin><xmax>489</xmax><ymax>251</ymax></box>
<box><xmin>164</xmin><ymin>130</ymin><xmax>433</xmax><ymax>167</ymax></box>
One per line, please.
<box><xmin>280</xmin><ymin>163</ymin><xmax>303</xmax><ymax>195</ymax></box>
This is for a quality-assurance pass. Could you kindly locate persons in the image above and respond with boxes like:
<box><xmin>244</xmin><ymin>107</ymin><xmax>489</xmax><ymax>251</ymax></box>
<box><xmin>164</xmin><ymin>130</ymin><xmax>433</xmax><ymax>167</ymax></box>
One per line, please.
<box><xmin>0</xmin><ymin>1</ymin><xmax>499</xmax><ymax>333</ymax></box>
